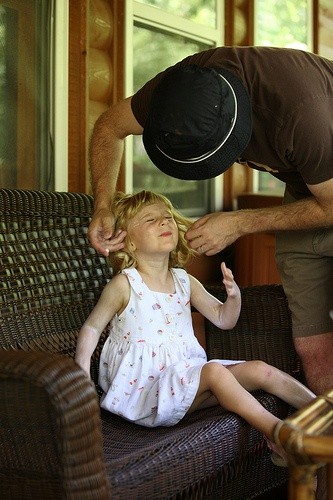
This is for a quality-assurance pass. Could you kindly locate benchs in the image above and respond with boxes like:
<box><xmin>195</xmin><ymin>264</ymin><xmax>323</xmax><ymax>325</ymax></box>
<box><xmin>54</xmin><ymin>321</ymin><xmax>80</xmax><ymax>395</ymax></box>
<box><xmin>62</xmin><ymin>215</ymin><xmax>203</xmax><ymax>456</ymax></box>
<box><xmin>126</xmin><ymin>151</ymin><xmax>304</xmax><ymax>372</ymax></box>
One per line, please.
<box><xmin>0</xmin><ymin>186</ymin><xmax>309</xmax><ymax>500</ymax></box>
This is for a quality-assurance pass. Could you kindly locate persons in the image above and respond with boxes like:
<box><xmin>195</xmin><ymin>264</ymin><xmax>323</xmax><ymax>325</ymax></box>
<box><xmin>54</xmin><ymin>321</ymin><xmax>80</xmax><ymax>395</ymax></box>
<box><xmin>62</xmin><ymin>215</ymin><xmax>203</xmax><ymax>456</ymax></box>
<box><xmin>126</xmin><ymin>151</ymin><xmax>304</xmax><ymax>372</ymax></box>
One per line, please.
<box><xmin>86</xmin><ymin>46</ymin><xmax>333</xmax><ymax>395</ymax></box>
<box><xmin>76</xmin><ymin>190</ymin><xmax>317</xmax><ymax>467</ymax></box>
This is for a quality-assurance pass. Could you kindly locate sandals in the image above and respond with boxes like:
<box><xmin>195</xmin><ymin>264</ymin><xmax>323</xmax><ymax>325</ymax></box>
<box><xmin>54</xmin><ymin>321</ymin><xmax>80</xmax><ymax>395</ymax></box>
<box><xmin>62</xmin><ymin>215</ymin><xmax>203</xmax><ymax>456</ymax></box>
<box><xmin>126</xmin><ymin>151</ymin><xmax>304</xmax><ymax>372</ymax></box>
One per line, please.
<box><xmin>263</xmin><ymin>425</ymin><xmax>293</xmax><ymax>468</ymax></box>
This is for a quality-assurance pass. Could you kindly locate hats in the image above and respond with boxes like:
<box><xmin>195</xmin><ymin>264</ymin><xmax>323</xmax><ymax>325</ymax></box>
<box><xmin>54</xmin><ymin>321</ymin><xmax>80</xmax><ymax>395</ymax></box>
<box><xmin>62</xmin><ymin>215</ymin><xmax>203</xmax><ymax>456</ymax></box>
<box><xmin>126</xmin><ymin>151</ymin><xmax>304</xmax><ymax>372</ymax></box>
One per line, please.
<box><xmin>142</xmin><ymin>65</ymin><xmax>252</xmax><ymax>181</ymax></box>
